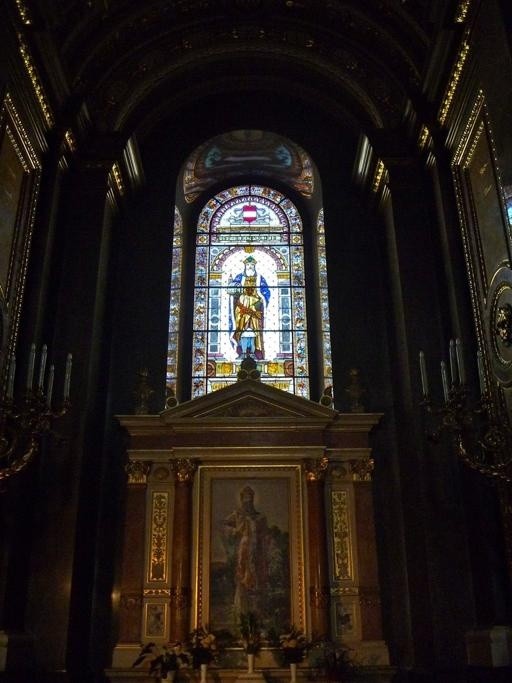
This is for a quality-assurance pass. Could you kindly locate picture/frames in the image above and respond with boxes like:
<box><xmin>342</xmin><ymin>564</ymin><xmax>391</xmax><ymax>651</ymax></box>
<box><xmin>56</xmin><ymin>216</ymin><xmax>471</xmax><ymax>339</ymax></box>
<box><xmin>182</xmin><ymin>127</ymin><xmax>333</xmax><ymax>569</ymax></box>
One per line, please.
<box><xmin>198</xmin><ymin>465</ymin><xmax>307</xmax><ymax>651</ymax></box>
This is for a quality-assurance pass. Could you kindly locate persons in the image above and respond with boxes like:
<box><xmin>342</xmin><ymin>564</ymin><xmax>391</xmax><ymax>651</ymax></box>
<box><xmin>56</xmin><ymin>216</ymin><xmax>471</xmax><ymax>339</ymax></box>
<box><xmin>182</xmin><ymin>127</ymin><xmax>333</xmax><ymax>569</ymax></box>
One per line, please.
<box><xmin>226</xmin><ymin>256</ymin><xmax>271</xmax><ymax>359</ymax></box>
<box><xmin>217</xmin><ymin>485</ymin><xmax>284</xmax><ymax>623</ymax></box>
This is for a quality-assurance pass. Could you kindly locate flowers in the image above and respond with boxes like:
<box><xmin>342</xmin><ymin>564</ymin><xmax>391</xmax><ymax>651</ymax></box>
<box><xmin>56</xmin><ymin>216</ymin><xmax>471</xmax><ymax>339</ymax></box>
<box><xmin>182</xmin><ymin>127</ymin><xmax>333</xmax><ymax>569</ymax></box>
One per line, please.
<box><xmin>133</xmin><ymin>624</ymin><xmax>355</xmax><ymax>683</ymax></box>
